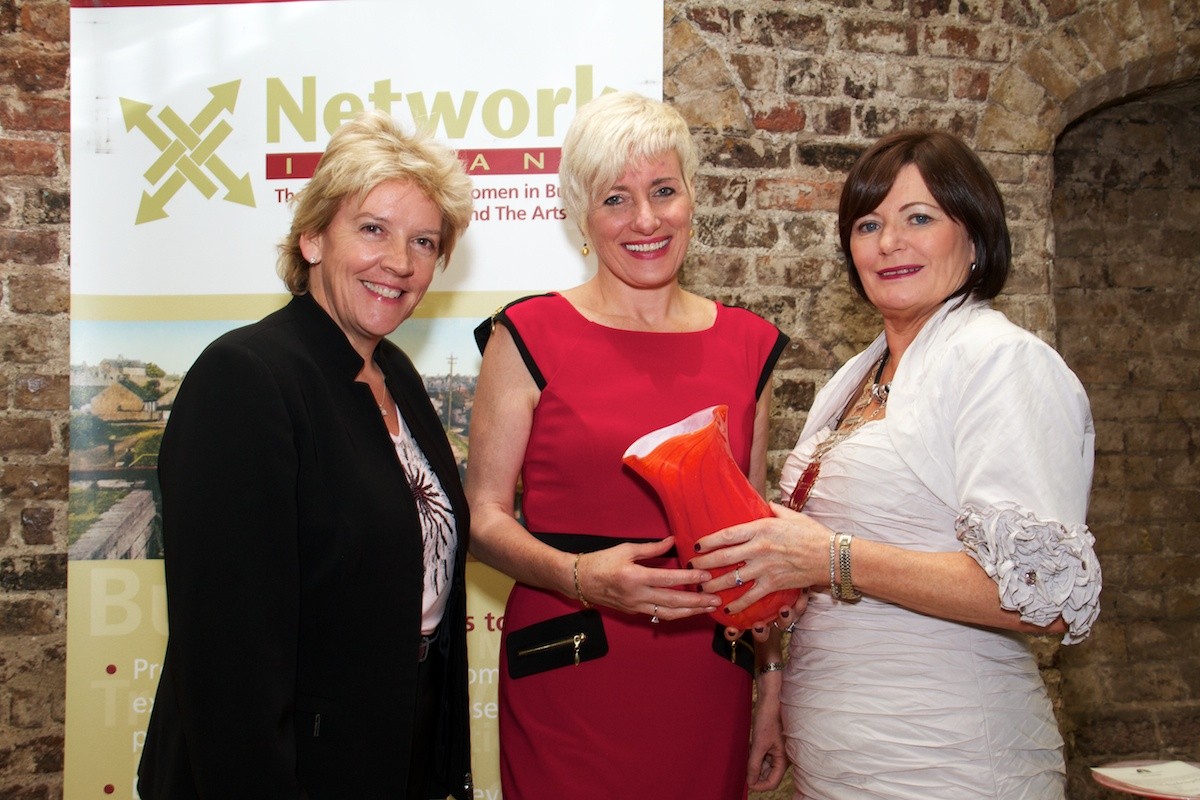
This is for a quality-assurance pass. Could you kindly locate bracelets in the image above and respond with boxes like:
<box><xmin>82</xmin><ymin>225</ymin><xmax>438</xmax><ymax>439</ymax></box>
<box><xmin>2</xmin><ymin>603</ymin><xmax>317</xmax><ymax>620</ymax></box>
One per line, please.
<box><xmin>829</xmin><ymin>532</ymin><xmax>842</xmax><ymax>598</ymax></box>
<box><xmin>574</xmin><ymin>553</ymin><xmax>594</xmax><ymax>608</ymax></box>
<box><xmin>758</xmin><ymin>662</ymin><xmax>784</xmax><ymax>674</ymax></box>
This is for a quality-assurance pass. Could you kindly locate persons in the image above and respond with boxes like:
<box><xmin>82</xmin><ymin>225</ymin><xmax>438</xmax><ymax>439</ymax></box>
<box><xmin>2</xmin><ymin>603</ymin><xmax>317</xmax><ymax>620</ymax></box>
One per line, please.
<box><xmin>464</xmin><ymin>91</ymin><xmax>789</xmax><ymax>800</ymax></box>
<box><xmin>137</xmin><ymin>106</ymin><xmax>474</xmax><ymax>799</ymax></box>
<box><xmin>686</xmin><ymin>128</ymin><xmax>1101</xmax><ymax>800</ymax></box>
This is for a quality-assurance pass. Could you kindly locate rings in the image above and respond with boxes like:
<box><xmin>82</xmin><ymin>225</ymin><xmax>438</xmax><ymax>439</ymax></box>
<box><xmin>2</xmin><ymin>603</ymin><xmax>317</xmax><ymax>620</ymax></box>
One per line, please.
<box><xmin>735</xmin><ymin>569</ymin><xmax>743</xmax><ymax>585</ymax></box>
<box><xmin>785</xmin><ymin>622</ymin><xmax>795</xmax><ymax>632</ymax></box>
<box><xmin>651</xmin><ymin>605</ymin><xmax>659</xmax><ymax>623</ymax></box>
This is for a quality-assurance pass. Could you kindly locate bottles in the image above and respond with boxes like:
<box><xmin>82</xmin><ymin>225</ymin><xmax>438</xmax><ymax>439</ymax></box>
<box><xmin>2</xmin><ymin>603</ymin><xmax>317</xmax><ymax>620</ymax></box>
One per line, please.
<box><xmin>620</xmin><ymin>405</ymin><xmax>803</xmax><ymax>631</ymax></box>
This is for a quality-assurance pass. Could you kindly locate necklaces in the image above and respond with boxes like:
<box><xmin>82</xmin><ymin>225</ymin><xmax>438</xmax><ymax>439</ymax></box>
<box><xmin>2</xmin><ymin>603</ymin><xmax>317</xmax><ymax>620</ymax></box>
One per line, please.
<box><xmin>373</xmin><ymin>359</ymin><xmax>388</xmax><ymax>416</ymax></box>
<box><xmin>789</xmin><ymin>349</ymin><xmax>892</xmax><ymax>513</ymax></box>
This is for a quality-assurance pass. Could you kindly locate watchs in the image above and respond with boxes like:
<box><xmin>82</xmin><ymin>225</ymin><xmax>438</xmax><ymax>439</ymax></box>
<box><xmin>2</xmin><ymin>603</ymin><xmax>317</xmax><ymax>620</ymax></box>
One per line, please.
<box><xmin>838</xmin><ymin>533</ymin><xmax>862</xmax><ymax>599</ymax></box>
